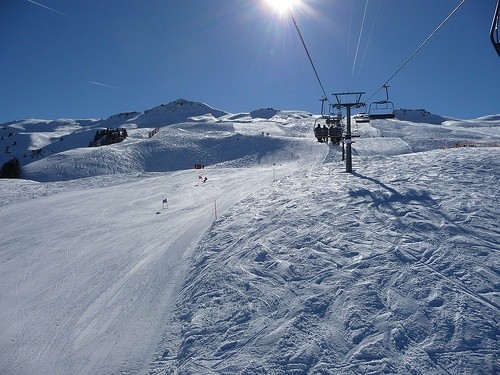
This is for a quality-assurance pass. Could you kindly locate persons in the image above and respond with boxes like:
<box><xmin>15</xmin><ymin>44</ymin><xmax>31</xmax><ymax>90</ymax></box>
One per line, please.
<box><xmin>321</xmin><ymin>125</ymin><xmax>328</xmax><ymax>144</ymax></box>
<box><xmin>328</xmin><ymin>126</ymin><xmax>344</xmax><ymax>145</ymax></box>
<box><xmin>314</xmin><ymin>124</ymin><xmax>323</xmax><ymax>143</ymax></box>
